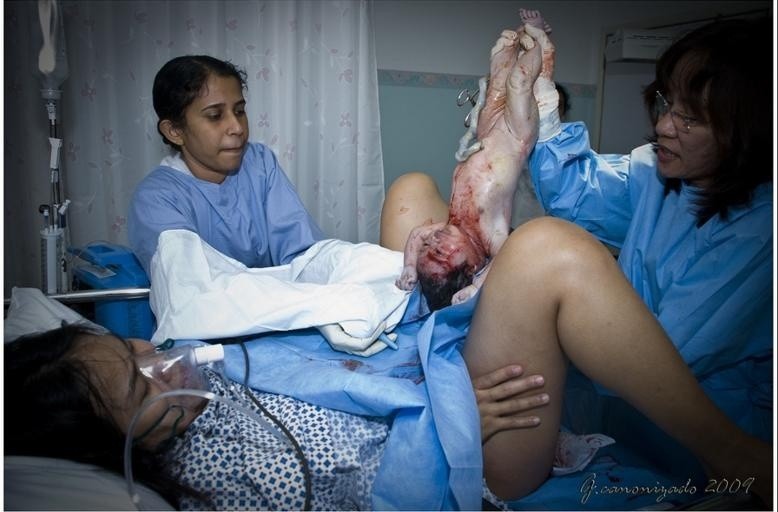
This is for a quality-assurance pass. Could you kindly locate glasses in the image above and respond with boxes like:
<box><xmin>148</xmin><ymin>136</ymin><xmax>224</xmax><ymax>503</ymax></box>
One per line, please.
<box><xmin>654</xmin><ymin>89</ymin><xmax>711</xmax><ymax>134</ymax></box>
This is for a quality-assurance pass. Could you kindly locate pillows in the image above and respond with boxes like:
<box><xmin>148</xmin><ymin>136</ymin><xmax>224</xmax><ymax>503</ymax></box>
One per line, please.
<box><xmin>3</xmin><ymin>284</ymin><xmax>176</xmax><ymax>509</ymax></box>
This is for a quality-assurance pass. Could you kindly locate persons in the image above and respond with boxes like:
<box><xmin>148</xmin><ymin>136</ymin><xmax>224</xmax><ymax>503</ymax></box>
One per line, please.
<box><xmin>490</xmin><ymin>10</ymin><xmax>774</xmax><ymax>509</ymax></box>
<box><xmin>509</xmin><ymin>80</ymin><xmax>572</xmax><ymax>228</ymax></box>
<box><xmin>128</xmin><ymin>52</ymin><xmax>398</xmax><ymax>358</ymax></box>
<box><xmin>5</xmin><ymin>172</ymin><xmax>771</xmax><ymax>511</ymax></box>
<box><xmin>395</xmin><ymin>8</ymin><xmax>553</xmax><ymax>307</ymax></box>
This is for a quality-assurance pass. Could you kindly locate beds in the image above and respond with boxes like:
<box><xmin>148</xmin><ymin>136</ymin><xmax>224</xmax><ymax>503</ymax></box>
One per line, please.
<box><xmin>3</xmin><ymin>284</ymin><xmax>774</xmax><ymax>511</ymax></box>
